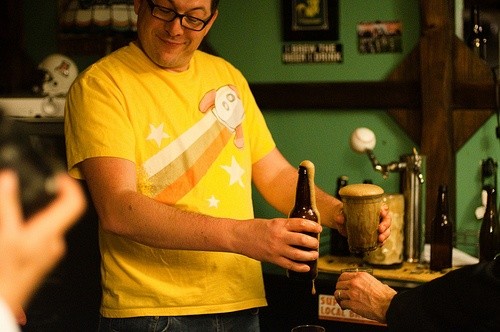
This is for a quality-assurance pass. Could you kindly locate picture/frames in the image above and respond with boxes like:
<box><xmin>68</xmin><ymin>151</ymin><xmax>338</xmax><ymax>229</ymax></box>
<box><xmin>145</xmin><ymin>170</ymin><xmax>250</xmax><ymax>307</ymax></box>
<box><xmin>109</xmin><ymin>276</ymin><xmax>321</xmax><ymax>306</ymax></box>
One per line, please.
<box><xmin>281</xmin><ymin>0</ymin><xmax>340</xmax><ymax>41</ymax></box>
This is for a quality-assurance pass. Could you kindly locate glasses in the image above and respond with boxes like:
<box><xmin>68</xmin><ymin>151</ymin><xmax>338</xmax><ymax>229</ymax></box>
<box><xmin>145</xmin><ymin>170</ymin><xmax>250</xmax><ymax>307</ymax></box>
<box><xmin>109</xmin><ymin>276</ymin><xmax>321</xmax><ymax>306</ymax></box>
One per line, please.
<box><xmin>148</xmin><ymin>0</ymin><xmax>212</xmax><ymax>30</ymax></box>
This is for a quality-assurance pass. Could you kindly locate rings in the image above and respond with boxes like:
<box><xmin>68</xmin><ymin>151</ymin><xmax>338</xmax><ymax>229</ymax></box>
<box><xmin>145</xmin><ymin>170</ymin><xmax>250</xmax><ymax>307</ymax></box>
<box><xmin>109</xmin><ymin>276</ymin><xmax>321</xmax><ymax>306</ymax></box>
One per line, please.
<box><xmin>339</xmin><ymin>290</ymin><xmax>342</xmax><ymax>301</ymax></box>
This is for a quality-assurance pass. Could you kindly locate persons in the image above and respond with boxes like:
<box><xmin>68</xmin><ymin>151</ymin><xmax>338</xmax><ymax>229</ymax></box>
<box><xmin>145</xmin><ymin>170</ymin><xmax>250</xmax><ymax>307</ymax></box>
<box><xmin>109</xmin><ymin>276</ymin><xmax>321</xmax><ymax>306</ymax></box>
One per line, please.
<box><xmin>64</xmin><ymin>0</ymin><xmax>392</xmax><ymax>332</ymax></box>
<box><xmin>334</xmin><ymin>256</ymin><xmax>500</xmax><ymax>332</ymax></box>
<box><xmin>0</xmin><ymin>170</ymin><xmax>88</xmax><ymax>332</ymax></box>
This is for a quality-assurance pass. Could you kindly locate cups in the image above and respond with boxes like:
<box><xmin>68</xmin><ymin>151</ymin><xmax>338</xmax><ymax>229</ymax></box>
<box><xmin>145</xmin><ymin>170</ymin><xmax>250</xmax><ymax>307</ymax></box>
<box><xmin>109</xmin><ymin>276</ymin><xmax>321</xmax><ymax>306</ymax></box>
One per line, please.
<box><xmin>363</xmin><ymin>194</ymin><xmax>407</xmax><ymax>268</ymax></box>
<box><xmin>340</xmin><ymin>267</ymin><xmax>373</xmax><ymax>278</ymax></box>
<box><xmin>290</xmin><ymin>325</ymin><xmax>325</xmax><ymax>332</ymax></box>
<box><xmin>340</xmin><ymin>190</ymin><xmax>385</xmax><ymax>254</ymax></box>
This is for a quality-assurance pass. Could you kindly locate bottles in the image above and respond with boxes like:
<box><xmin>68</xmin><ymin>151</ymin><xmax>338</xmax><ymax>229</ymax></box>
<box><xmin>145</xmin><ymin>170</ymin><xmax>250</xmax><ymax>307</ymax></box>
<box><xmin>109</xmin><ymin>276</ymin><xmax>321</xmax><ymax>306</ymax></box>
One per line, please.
<box><xmin>329</xmin><ymin>175</ymin><xmax>350</xmax><ymax>256</ymax></box>
<box><xmin>478</xmin><ymin>189</ymin><xmax>500</xmax><ymax>282</ymax></box>
<box><xmin>287</xmin><ymin>163</ymin><xmax>318</xmax><ymax>281</ymax></box>
<box><xmin>430</xmin><ymin>184</ymin><xmax>454</xmax><ymax>271</ymax></box>
